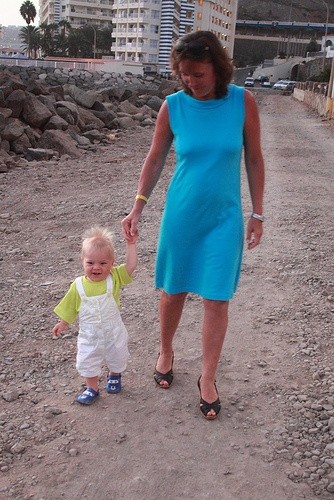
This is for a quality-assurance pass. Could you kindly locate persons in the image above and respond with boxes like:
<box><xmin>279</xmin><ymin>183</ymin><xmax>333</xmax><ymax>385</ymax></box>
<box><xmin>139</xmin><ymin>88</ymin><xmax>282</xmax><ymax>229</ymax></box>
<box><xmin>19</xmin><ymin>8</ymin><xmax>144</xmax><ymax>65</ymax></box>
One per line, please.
<box><xmin>51</xmin><ymin>227</ymin><xmax>140</xmax><ymax>405</ymax></box>
<box><xmin>121</xmin><ymin>30</ymin><xmax>265</xmax><ymax>419</ymax></box>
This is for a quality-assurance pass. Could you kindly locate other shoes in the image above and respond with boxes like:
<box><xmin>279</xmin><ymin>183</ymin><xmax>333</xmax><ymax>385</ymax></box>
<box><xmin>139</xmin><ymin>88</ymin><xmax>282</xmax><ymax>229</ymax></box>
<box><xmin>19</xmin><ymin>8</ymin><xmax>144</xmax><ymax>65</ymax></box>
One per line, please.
<box><xmin>76</xmin><ymin>388</ymin><xmax>100</xmax><ymax>404</ymax></box>
<box><xmin>106</xmin><ymin>372</ymin><xmax>121</xmax><ymax>393</ymax></box>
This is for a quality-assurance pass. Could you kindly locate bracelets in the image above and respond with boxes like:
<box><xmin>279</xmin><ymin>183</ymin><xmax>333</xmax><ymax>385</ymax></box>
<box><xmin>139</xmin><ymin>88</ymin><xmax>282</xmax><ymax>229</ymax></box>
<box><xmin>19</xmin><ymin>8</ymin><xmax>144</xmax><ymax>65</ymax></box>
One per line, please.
<box><xmin>251</xmin><ymin>213</ymin><xmax>265</xmax><ymax>222</ymax></box>
<box><xmin>135</xmin><ymin>194</ymin><xmax>148</xmax><ymax>204</ymax></box>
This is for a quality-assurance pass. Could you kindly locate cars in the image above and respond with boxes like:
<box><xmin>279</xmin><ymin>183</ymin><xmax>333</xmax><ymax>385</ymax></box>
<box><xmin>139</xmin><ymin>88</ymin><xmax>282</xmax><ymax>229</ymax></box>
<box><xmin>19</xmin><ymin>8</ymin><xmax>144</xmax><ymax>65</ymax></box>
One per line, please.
<box><xmin>244</xmin><ymin>76</ymin><xmax>254</xmax><ymax>86</ymax></box>
<box><xmin>272</xmin><ymin>79</ymin><xmax>297</xmax><ymax>91</ymax></box>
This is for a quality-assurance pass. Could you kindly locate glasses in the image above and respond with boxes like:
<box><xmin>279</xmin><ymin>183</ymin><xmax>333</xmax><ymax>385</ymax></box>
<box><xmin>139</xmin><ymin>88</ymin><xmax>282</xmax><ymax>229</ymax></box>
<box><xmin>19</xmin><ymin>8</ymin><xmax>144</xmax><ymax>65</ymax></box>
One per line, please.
<box><xmin>175</xmin><ymin>39</ymin><xmax>210</xmax><ymax>53</ymax></box>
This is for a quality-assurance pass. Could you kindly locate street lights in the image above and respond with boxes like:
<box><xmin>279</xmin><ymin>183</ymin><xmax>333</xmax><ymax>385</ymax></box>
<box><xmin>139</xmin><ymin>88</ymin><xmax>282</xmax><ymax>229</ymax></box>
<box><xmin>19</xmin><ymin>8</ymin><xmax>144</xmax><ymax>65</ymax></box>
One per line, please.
<box><xmin>85</xmin><ymin>23</ymin><xmax>97</xmax><ymax>71</ymax></box>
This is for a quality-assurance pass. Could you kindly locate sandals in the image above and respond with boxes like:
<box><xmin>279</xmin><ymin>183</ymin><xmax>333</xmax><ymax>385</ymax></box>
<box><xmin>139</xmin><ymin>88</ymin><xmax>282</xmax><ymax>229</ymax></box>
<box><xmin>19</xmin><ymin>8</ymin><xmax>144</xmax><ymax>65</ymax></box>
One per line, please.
<box><xmin>153</xmin><ymin>352</ymin><xmax>175</xmax><ymax>389</ymax></box>
<box><xmin>196</xmin><ymin>374</ymin><xmax>221</xmax><ymax>419</ymax></box>
